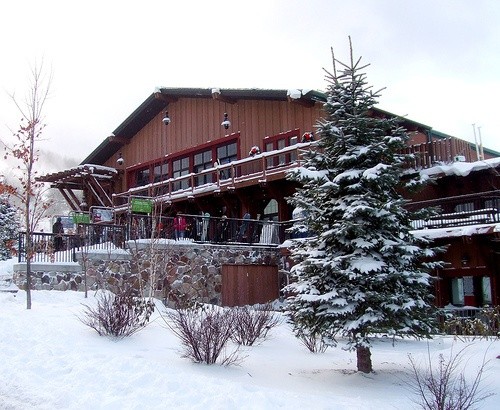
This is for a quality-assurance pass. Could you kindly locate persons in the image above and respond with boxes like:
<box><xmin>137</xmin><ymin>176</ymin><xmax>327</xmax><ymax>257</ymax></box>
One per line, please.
<box><xmin>174</xmin><ymin>212</ymin><xmax>186</xmax><ymax>240</ymax></box>
<box><xmin>218</xmin><ymin>215</ymin><xmax>228</xmax><ymax>241</ymax></box>
<box><xmin>53</xmin><ymin>217</ymin><xmax>64</xmax><ymax>252</ymax></box>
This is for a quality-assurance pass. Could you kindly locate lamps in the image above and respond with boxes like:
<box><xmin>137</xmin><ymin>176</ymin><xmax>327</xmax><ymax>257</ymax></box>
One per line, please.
<box><xmin>115</xmin><ymin>151</ymin><xmax>124</xmax><ymax>165</ymax></box>
<box><xmin>162</xmin><ymin>111</ymin><xmax>170</xmax><ymax>126</ymax></box>
<box><xmin>221</xmin><ymin>101</ymin><xmax>231</xmax><ymax>130</ymax></box>
